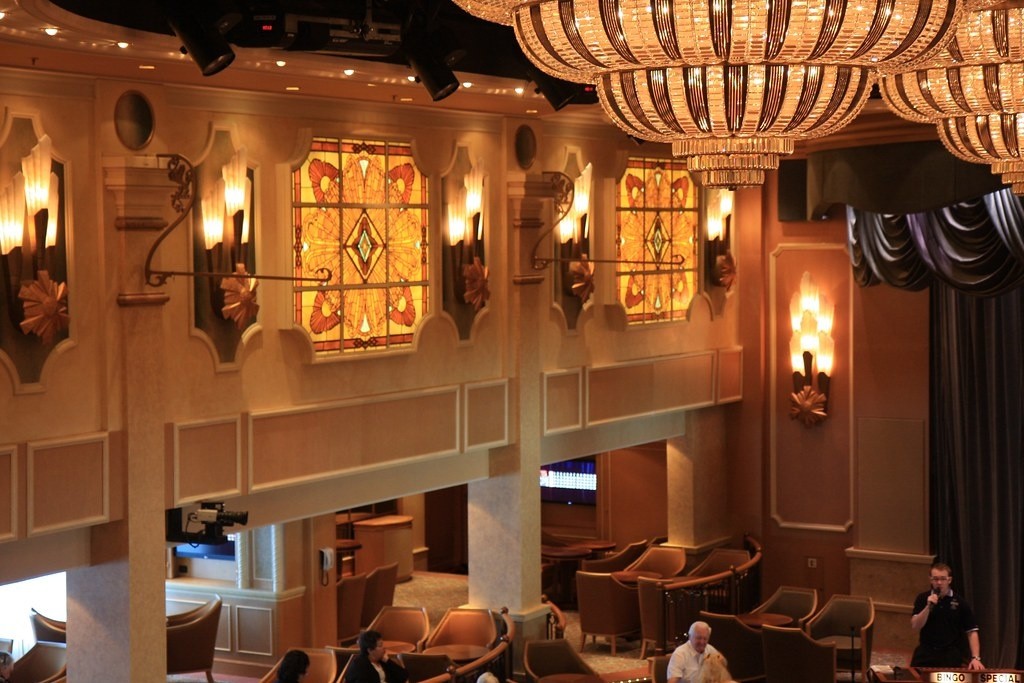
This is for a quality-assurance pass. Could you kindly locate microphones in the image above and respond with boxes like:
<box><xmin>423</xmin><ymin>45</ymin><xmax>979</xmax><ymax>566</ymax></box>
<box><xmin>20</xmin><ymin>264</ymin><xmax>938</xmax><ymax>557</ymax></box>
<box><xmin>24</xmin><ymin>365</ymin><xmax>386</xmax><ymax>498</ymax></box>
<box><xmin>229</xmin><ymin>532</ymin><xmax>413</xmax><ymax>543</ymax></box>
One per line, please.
<box><xmin>930</xmin><ymin>588</ymin><xmax>941</xmax><ymax>609</ymax></box>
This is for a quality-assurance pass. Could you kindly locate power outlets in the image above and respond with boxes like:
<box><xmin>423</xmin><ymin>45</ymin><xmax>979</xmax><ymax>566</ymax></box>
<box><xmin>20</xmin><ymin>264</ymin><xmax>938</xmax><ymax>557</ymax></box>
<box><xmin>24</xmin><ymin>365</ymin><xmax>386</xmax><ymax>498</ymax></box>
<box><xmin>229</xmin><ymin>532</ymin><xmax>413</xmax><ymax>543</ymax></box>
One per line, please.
<box><xmin>807</xmin><ymin>558</ymin><xmax>817</xmax><ymax>568</ymax></box>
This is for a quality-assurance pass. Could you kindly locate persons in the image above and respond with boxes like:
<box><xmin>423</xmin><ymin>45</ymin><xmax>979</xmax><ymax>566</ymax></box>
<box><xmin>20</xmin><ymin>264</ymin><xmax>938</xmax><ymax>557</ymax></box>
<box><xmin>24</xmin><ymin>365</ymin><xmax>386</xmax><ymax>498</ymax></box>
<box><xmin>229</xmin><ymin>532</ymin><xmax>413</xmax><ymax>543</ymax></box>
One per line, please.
<box><xmin>910</xmin><ymin>562</ymin><xmax>986</xmax><ymax>670</ymax></box>
<box><xmin>345</xmin><ymin>630</ymin><xmax>408</xmax><ymax>683</ymax></box>
<box><xmin>667</xmin><ymin>621</ymin><xmax>725</xmax><ymax>683</ymax></box>
<box><xmin>0</xmin><ymin>652</ymin><xmax>14</xmax><ymax>683</ymax></box>
<box><xmin>271</xmin><ymin>650</ymin><xmax>310</xmax><ymax>683</ymax></box>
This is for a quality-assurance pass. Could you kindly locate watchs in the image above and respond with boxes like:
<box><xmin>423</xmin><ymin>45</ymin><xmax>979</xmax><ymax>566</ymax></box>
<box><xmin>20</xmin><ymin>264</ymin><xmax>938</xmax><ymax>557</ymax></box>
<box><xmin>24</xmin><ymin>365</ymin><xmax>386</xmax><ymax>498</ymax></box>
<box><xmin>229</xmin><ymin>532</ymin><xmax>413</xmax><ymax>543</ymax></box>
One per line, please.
<box><xmin>971</xmin><ymin>656</ymin><xmax>981</xmax><ymax>661</ymax></box>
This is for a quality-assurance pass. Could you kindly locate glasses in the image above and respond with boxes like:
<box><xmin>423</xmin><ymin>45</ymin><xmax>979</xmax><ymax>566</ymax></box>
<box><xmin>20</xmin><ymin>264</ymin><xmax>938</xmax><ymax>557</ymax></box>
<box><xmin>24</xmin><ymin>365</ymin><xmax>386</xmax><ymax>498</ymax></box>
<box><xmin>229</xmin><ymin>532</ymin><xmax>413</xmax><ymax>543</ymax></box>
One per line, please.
<box><xmin>931</xmin><ymin>576</ymin><xmax>947</xmax><ymax>581</ymax></box>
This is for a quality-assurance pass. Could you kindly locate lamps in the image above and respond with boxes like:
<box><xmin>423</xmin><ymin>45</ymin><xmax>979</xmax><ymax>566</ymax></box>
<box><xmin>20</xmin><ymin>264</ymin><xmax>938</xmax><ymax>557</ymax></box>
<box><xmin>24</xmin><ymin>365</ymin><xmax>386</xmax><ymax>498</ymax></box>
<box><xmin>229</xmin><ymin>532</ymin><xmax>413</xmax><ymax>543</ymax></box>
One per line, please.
<box><xmin>449</xmin><ymin>151</ymin><xmax>491</xmax><ymax>314</ymax></box>
<box><xmin>559</xmin><ymin>162</ymin><xmax>597</xmax><ymax>304</ymax></box>
<box><xmin>705</xmin><ymin>192</ymin><xmax>738</xmax><ymax>291</ymax></box>
<box><xmin>401</xmin><ymin>0</ymin><xmax>1024</xmax><ymax>198</ymax></box>
<box><xmin>162</xmin><ymin>1</ymin><xmax>238</xmax><ymax>77</ymax></box>
<box><xmin>0</xmin><ymin>134</ymin><xmax>72</xmax><ymax>337</ymax></box>
<box><xmin>202</xmin><ymin>145</ymin><xmax>264</xmax><ymax>328</ymax></box>
<box><xmin>786</xmin><ymin>270</ymin><xmax>839</xmax><ymax>427</ymax></box>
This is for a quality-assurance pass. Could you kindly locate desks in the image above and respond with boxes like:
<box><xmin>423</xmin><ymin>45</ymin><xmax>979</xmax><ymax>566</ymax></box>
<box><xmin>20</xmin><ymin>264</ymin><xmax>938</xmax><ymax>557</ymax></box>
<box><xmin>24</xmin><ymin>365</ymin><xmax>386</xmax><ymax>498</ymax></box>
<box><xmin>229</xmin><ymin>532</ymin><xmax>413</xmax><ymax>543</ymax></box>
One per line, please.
<box><xmin>541</xmin><ymin>548</ymin><xmax>593</xmax><ymax>610</ymax></box>
<box><xmin>348</xmin><ymin>641</ymin><xmax>416</xmax><ymax>655</ymax></box>
<box><xmin>566</xmin><ymin>538</ymin><xmax>617</xmax><ymax>551</ymax></box>
<box><xmin>336</xmin><ymin>539</ymin><xmax>362</xmax><ymax>582</ymax></box>
<box><xmin>738</xmin><ymin>614</ymin><xmax>794</xmax><ymax>626</ymax></box>
<box><xmin>612</xmin><ymin>570</ymin><xmax>663</xmax><ymax>583</ymax></box>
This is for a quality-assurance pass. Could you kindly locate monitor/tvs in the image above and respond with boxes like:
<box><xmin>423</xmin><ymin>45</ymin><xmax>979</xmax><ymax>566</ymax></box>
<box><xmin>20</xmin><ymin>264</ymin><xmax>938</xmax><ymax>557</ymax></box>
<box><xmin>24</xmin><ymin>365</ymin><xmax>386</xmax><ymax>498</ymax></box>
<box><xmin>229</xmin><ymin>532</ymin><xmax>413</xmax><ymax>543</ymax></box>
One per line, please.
<box><xmin>175</xmin><ymin>533</ymin><xmax>235</xmax><ymax>561</ymax></box>
<box><xmin>539</xmin><ymin>455</ymin><xmax>596</xmax><ymax>506</ymax></box>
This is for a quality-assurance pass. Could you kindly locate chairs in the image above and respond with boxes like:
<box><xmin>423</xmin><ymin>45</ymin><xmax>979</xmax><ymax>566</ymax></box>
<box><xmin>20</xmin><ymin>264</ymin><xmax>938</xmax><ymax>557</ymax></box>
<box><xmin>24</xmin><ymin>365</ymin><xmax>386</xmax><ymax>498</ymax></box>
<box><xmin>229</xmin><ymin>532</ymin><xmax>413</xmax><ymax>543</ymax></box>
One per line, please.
<box><xmin>805</xmin><ymin>594</ymin><xmax>875</xmax><ymax>683</ymax></box>
<box><xmin>748</xmin><ymin>585</ymin><xmax>818</xmax><ymax>629</ymax></box>
<box><xmin>622</xmin><ymin>545</ymin><xmax>686</xmax><ymax>579</ymax></box>
<box><xmin>577</xmin><ymin>571</ymin><xmax>640</xmax><ymax>656</ymax></box>
<box><xmin>10</xmin><ymin>641</ymin><xmax>67</xmax><ymax>683</ymax></box>
<box><xmin>760</xmin><ymin>623</ymin><xmax>837</xmax><ymax>683</ymax></box>
<box><xmin>260</xmin><ymin>647</ymin><xmax>338</xmax><ymax>683</ymax></box>
<box><xmin>698</xmin><ymin>611</ymin><xmax>766</xmax><ymax>683</ymax></box>
<box><xmin>580</xmin><ymin>540</ymin><xmax>648</xmax><ymax>572</ymax></box>
<box><xmin>339</xmin><ymin>606</ymin><xmax>432</xmax><ymax>656</ymax></box>
<box><xmin>423</xmin><ymin>608</ymin><xmax>498</xmax><ymax>662</ymax></box>
<box><xmin>522</xmin><ymin>638</ymin><xmax>600</xmax><ymax>683</ymax></box>
<box><xmin>166</xmin><ymin>593</ymin><xmax>223</xmax><ymax>683</ymax></box>
<box><xmin>336</xmin><ymin>573</ymin><xmax>366</xmax><ymax>646</ymax></box>
<box><xmin>361</xmin><ymin>561</ymin><xmax>400</xmax><ymax>628</ymax></box>
<box><xmin>30</xmin><ymin>607</ymin><xmax>66</xmax><ymax>643</ymax></box>
